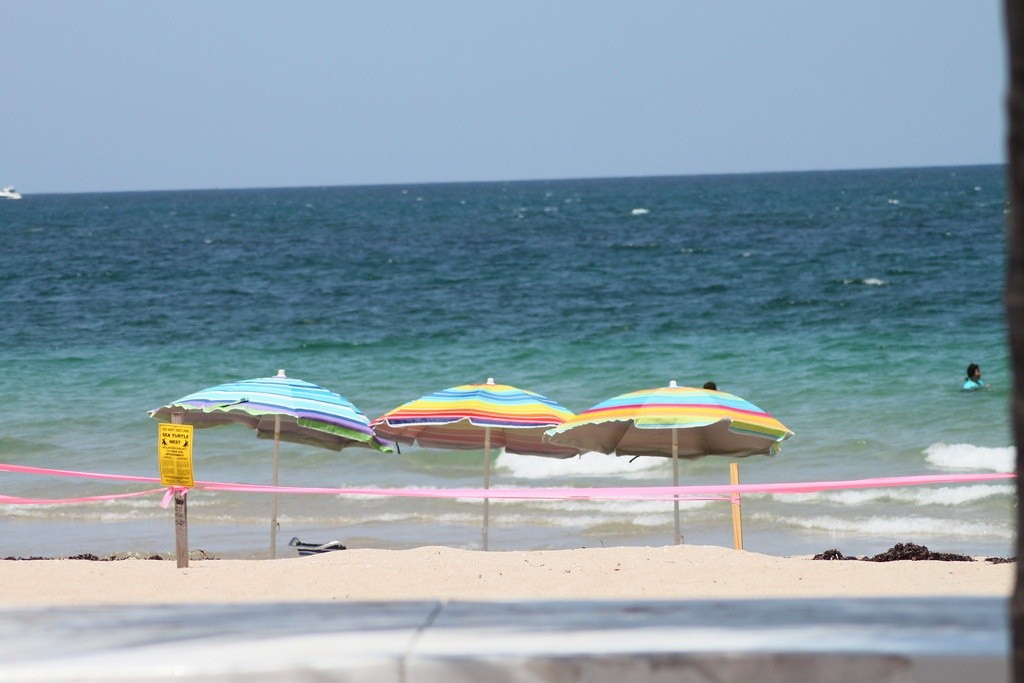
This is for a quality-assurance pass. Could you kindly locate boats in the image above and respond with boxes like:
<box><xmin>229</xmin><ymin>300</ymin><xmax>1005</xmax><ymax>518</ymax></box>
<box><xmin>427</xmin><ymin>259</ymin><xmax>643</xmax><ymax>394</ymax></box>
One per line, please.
<box><xmin>0</xmin><ymin>187</ymin><xmax>22</xmax><ymax>200</ymax></box>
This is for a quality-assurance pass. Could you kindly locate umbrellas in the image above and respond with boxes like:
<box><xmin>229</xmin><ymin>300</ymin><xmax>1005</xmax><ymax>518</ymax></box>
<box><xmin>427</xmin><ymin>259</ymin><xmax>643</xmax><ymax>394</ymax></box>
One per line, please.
<box><xmin>146</xmin><ymin>368</ymin><xmax>393</xmax><ymax>558</ymax></box>
<box><xmin>541</xmin><ymin>381</ymin><xmax>794</xmax><ymax>545</ymax></box>
<box><xmin>368</xmin><ymin>378</ymin><xmax>580</xmax><ymax>550</ymax></box>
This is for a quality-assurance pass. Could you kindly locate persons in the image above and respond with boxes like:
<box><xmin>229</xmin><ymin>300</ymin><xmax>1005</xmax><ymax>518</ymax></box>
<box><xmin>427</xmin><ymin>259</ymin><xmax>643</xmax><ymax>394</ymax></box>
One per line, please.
<box><xmin>702</xmin><ymin>381</ymin><xmax>719</xmax><ymax>391</ymax></box>
<box><xmin>957</xmin><ymin>360</ymin><xmax>992</xmax><ymax>395</ymax></box>
